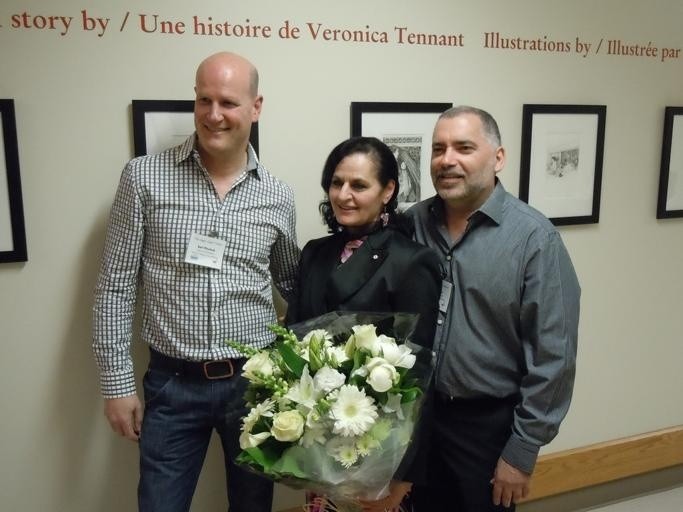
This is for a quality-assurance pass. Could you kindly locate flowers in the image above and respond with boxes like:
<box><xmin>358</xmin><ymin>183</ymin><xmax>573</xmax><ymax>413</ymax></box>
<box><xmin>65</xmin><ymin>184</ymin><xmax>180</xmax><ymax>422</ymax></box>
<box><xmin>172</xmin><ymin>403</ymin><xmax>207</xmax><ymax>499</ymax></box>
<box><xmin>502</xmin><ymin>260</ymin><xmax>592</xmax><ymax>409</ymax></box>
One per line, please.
<box><xmin>227</xmin><ymin>312</ymin><xmax>429</xmax><ymax>500</ymax></box>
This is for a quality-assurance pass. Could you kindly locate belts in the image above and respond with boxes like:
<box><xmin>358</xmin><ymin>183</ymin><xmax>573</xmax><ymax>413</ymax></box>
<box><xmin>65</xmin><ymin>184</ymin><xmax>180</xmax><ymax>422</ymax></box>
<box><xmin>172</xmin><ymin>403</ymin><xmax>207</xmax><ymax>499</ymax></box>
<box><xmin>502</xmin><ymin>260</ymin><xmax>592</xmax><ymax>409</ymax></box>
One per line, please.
<box><xmin>146</xmin><ymin>347</ymin><xmax>249</xmax><ymax>381</ymax></box>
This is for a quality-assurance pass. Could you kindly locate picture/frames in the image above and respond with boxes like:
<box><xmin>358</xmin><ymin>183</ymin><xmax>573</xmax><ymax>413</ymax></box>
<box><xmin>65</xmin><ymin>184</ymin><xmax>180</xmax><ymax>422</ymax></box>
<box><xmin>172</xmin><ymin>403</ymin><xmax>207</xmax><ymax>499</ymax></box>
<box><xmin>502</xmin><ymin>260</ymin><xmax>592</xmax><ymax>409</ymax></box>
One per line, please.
<box><xmin>128</xmin><ymin>99</ymin><xmax>259</xmax><ymax>180</ymax></box>
<box><xmin>518</xmin><ymin>104</ymin><xmax>608</xmax><ymax>227</ymax></box>
<box><xmin>350</xmin><ymin>100</ymin><xmax>451</xmax><ymax>212</ymax></box>
<box><xmin>0</xmin><ymin>97</ymin><xmax>26</xmax><ymax>262</ymax></box>
<box><xmin>655</xmin><ymin>105</ymin><xmax>682</xmax><ymax>219</ymax></box>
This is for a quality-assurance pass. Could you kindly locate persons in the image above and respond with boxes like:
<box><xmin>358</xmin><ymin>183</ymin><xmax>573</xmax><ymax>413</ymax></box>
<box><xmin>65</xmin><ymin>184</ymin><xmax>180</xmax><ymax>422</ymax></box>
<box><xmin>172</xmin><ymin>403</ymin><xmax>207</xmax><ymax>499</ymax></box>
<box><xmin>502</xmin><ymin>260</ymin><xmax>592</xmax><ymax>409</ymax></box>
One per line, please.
<box><xmin>92</xmin><ymin>54</ymin><xmax>304</xmax><ymax>512</ymax></box>
<box><xmin>276</xmin><ymin>137</ymin><xmax>447</xmax><ymax>512</ymax></box>
<box><xmin>400</xmin><ymin>105</ymin><xmax>582</xmax><ymax>512</ymax></box>
